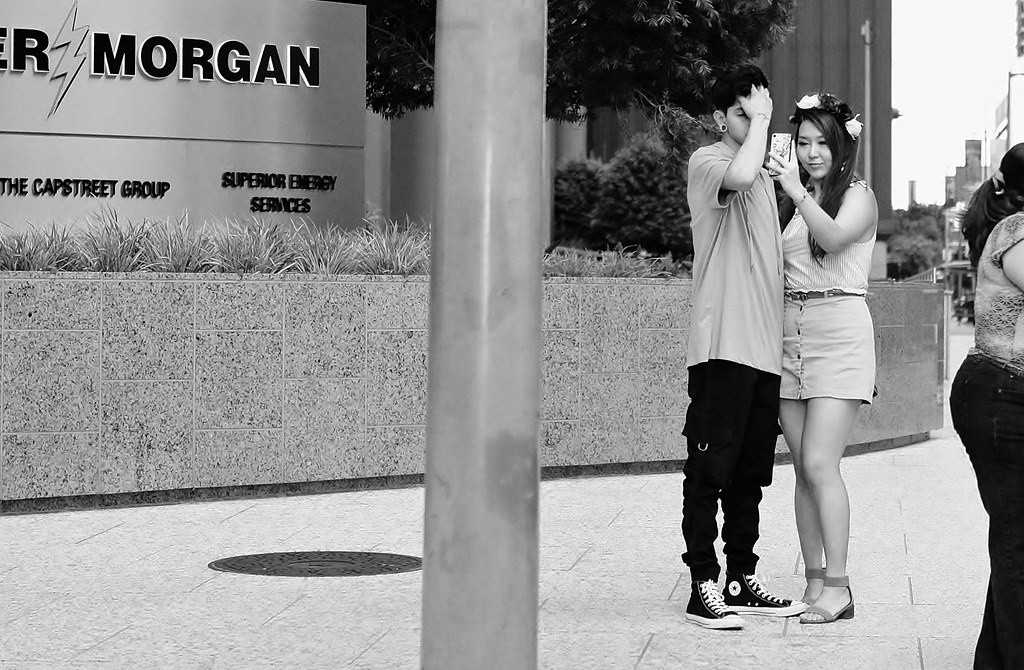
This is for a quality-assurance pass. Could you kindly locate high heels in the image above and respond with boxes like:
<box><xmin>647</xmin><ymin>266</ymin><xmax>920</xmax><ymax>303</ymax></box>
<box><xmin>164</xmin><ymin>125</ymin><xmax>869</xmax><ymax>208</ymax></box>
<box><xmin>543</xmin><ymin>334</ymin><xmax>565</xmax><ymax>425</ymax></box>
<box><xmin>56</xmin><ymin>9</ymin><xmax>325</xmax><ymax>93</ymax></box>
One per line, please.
<box><xmin>800</xmin><ymin>568</ymin><xmax>854</xmax><ymax>624</ymax></box>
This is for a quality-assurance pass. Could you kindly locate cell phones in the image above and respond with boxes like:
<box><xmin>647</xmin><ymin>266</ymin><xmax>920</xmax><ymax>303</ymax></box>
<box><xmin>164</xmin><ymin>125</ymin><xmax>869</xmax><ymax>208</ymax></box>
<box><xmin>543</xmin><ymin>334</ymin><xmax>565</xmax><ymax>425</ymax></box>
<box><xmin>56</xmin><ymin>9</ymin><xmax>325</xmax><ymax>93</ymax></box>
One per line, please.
<box><xmin>768</xmin><ymin>133</ymin><xmax>793</xmax><ymax>176</ymax></box>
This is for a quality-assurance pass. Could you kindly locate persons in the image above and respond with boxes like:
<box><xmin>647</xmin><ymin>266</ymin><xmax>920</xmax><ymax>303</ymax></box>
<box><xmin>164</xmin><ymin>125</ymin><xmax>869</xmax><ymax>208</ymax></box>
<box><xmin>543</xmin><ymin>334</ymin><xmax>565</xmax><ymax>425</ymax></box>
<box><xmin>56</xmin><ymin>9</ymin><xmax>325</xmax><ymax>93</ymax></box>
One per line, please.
<box><xmin>764</xmin><ymin>93</ymin><xmax>878</xmax><ymax>624</ymax></box>
<box><xmin>682</xmin><ymin>65</ymin><xmax>806</xmax><ymax>629</ymax></box>
<box><xmin>950</xmin><ymin>142</ymin><xmax>1024</xmax><ymax>670</ymax></box>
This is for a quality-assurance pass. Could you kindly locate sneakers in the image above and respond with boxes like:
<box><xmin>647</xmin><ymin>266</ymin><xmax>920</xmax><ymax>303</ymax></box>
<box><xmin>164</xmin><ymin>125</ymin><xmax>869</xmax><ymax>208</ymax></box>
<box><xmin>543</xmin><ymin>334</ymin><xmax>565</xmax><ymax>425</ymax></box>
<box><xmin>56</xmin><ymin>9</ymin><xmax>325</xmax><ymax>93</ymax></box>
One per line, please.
<box><xmin>721</xmin><ymin>571</ymin><xmax>808</xmax><ymax>617</ymax></box>
<box><xmin>686</xmin><ymin>580</ymin><xmax>746</xmax><ymax>629</ymax></box>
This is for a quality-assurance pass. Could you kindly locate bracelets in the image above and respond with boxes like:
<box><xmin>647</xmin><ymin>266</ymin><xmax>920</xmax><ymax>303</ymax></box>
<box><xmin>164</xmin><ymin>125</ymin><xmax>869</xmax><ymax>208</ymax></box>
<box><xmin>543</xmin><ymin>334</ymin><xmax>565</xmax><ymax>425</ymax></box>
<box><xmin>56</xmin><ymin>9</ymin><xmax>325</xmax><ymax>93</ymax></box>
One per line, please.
<box><xmin>793</xmin><ymin>189</ymin><xmax>806</xmax><ymax>206</ymax></box>
